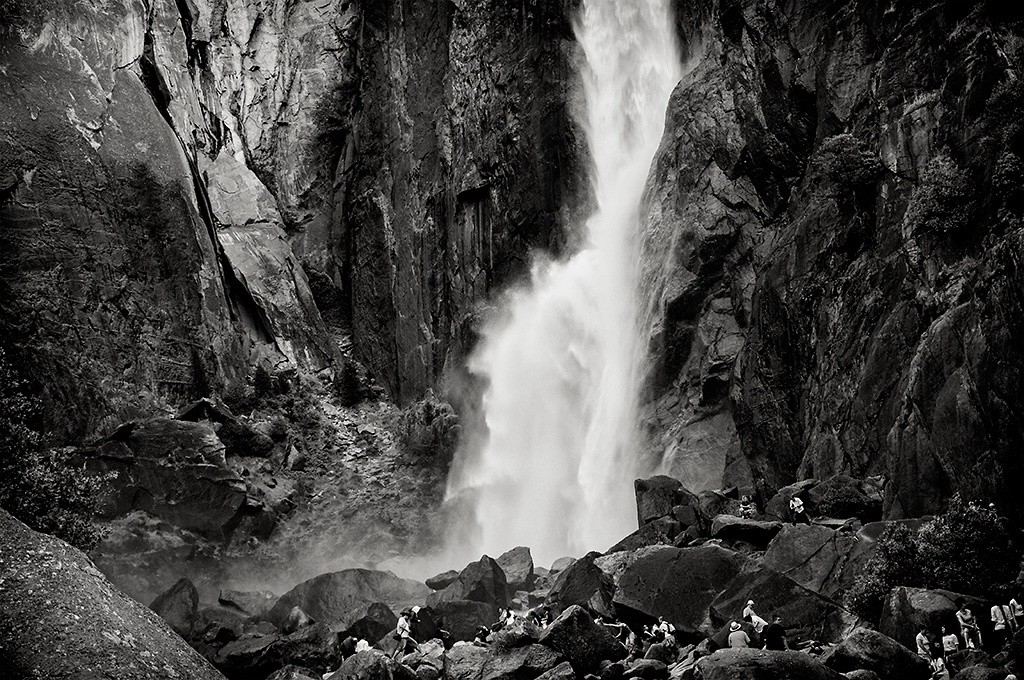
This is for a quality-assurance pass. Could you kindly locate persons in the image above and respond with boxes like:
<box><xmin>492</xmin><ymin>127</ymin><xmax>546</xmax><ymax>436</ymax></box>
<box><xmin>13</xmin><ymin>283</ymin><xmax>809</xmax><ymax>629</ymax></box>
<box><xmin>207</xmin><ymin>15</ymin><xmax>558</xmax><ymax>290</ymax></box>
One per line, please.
<box><xmin>928</xmin><ymin>649</ymin><xmax>949</xmax><ymax>680</ymax></box>
<box><xmin>788</xmin><ymin>495</ymin><xmax>811</xmax><ymax>527</ymax></box>
<box><xmin>742</xmin><ymin>600</ymin><xmax>759</xmax><ymax>628</ymax></box>
<box><xmin>1004</xmin><ymin>667</ymin><xmax>1017</xmax><ymax>680</ymax></box>
<box><xmin>339</xmin><ymin>633</ymin><xmax>369</xmax><ymax>660</ymax></box>
<box><xmin>955</xmin><ymin>597</ymin><xmax>983</xmax><ymax>649</ymax></box>
<box><xmin>763</xmin><ymin>614</ymin><xmax>789</xmax><ymax>651</ymax></box>
<box><xmin>390</xmin><ymin>607</ymin><xmax>419</xmax><ymax>661</ymax></box>
<box><xmin>474</xmin><ymin>606</ymin><xmax>553</xmax><ymax>646</ymax></box>
<box><xmin>594</xmin><ymin>615</ymin><xmax>676</xmax><ymax>653</ymax></box>
<box><xmin>728</xmin><ymin>622</ymin><xmax>751</xmax><ymax>648</ymax></box>
<box><xmin>990</xmin><ymin>592</ymin><xmax>1020</xmax><ymax>641</ymax></box>
<box><xmin>940</xmin><ymin>624</ymin><xmax>959</xmax><ymax>663</ymax></box>
<box><xmin>1009</xmin><ymin>586</ymin><xmax>1024</xmax><ymax>631</ymax></box>
<box><xmin>916</xmin><ymin>624</ymin><xmax>931</xmax><ymax>664</ymax></box>
